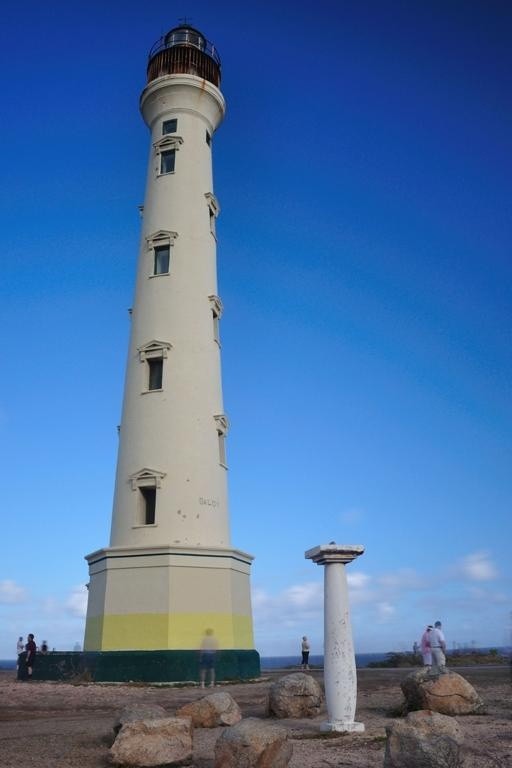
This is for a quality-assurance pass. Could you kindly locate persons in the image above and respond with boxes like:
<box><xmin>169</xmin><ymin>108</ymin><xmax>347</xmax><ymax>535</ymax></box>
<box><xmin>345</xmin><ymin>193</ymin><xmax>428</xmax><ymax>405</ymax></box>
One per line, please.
<box><xmin>300</xmin><ymin>636</ymin><xmax>310</xmax><ymax>671</ymax></box>
<box><xmin>17</xmin><ymin>633</ymin><xmax>48</xmax><ymax>681</ymax></box>
<box><xmin>199</xmin><ymin>627</ymin><xmax>218</xmax><ymax>690</ymax></box>
<box><xmin>413</xmin><ymin>641</ymin><xmax>419</xmax><ymax>654</ymax></box>
<box><xmin>420</xmin><ymin>621</ymin><xmax>448</xmax><ymax>667</ymax></box>
